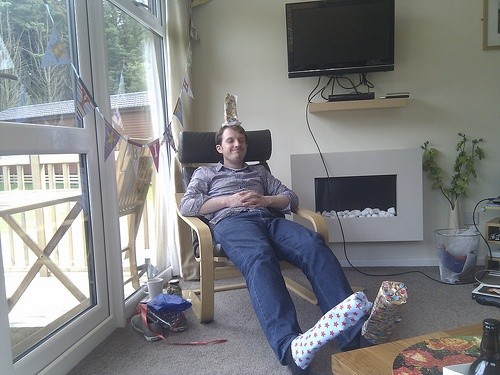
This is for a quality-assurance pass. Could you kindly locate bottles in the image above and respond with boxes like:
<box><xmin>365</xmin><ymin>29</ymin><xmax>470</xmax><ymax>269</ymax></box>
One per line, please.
<box><xmin>467</xmin><ymin>317</ymin><xmax>500</xmax><ymax>375</ymax></box>
<box><xmin>166</xmin><ymin>279</ymin><xmax>182</xmax><ymax>298</ymax></box>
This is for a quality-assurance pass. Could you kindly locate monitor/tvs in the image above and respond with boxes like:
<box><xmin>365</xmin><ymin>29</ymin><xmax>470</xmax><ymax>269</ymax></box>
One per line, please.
<box><xmin>284</xmin><ymin>0</ymin><xmax>396</xmax><ymax>78</ymax></box>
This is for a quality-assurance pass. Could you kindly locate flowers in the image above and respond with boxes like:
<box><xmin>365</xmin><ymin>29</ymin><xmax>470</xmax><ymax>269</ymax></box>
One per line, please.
<box><xmin>419</xmin><ymin>132</ymin><xmax>486</xmax><ymax>210</ymax></box>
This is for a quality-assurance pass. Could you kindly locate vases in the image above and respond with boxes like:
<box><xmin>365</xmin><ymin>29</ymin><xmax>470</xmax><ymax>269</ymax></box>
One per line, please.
<box><xmin>448</xmin><ymin>199</ymin><xmax>460</xmax><ymax>230</ymax></box>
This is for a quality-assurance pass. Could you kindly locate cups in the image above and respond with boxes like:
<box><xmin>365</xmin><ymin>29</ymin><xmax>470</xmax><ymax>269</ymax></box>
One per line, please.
<box><xmin>141</xmin><ymin>278</ymin><xmax>164</xmax><ymax>299</ymax></box>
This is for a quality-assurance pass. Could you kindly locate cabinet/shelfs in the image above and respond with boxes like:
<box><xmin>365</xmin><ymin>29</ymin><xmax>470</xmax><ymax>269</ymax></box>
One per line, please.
<box><xmin>483</xmin><ymin>201</ymin><xmax>500</xmax><ymax>271</ymax></box>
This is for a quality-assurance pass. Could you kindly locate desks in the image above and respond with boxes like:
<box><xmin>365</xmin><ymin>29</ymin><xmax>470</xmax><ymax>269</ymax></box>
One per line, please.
<box><xmin>331</xmin><ymin>320</ymin><xmax>484</xmax><ymax>375</ymax></box>
<box><xmin>0</xmin><ymin>187</ymin><xmax>88</xmax><ymax>313</ymax></box>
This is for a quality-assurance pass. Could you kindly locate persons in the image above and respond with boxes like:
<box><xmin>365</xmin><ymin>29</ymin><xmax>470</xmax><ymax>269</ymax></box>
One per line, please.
<box><xmin>179</xmin><ymin>123</ymin><xmax>407</xmax><ymax>375</ymax></box>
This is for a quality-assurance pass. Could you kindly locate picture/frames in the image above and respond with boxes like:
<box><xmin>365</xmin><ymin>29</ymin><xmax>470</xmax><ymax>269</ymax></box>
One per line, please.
<box><xmin>483</xmin><ymin>0</ymin><xmax>500</xmax><ymax>50</ymax></box>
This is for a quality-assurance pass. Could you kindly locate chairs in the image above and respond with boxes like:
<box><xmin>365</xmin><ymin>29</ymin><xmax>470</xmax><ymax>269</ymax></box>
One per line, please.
<box><xmin>172</xmin><ymin>129</ymin><xmax>329</xmax><ymax>325</ymax></box>
<box><xmin>115</xmin><ymin>135</ymin><xmax>154</xmax><ymax>290</ymax></box>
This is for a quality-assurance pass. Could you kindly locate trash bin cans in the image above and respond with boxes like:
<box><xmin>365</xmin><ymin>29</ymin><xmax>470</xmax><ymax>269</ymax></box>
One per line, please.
<box><xmin>434</xmin><ymin>229</ymin><xmax>481</xmax><ymax>284</ymax></box>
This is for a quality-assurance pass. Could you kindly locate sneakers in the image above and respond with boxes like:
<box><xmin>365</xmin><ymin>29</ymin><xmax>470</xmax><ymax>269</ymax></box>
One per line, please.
<box><xmin>162</xmin><ymin>315</ymin><xmax>188</xmax><ymax>331</ymax></box>
<box><xmin>131</xmin><ymin>314</ymin><xmax>163</xmax><ymax>341</ymax></box>
